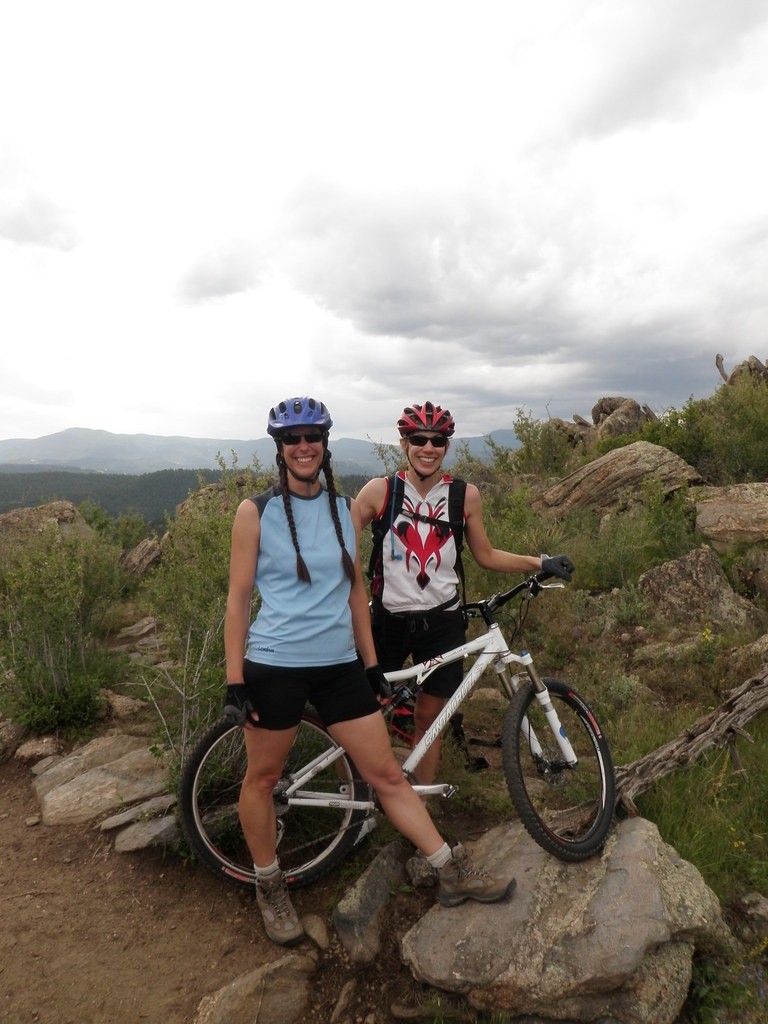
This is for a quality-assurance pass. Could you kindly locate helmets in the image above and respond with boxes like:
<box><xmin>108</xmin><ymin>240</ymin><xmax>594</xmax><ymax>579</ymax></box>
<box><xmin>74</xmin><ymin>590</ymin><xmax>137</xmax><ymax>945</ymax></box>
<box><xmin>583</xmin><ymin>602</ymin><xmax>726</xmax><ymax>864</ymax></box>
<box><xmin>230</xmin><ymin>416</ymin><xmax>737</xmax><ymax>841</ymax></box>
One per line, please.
<box><xmin>397</xmin><ymin>401</ymin><xmax>455</xmax><ymax>436</ymax></box>
<box><xmin>267</xmin><ymin>396</ymin><xmax>333</xmax><ymax>435</ymax></box>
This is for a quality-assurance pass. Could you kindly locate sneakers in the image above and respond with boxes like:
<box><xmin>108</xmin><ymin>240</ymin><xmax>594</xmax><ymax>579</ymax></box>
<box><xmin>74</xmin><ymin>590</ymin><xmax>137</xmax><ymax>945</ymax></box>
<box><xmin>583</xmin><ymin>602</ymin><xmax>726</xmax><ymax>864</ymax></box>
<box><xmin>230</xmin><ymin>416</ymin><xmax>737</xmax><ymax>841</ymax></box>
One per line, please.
<box><xmin>255</xmin><ymin>868</ymin><xmax>304</xmax><ymax>947</ymax></box>
<box><xmin>436</xmin><ymin>843</ymin><xmax>517</xmax><ymax>906</ymax></box>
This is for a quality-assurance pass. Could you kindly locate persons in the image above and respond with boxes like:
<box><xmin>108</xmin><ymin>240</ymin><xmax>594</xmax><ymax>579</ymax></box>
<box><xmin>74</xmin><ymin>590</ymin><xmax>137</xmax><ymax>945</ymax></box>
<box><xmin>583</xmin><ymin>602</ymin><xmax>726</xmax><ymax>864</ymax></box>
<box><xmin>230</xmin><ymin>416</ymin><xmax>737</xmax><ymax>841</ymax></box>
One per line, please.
<box><xmin>223</xmin><ymin>397</ymin><xmax>518</xmax><ymax>946</ymax></box>
<box><xmin>353</xmin><ymin>401</ymin><xmax>574</xmax><ymax>856</ymax></box>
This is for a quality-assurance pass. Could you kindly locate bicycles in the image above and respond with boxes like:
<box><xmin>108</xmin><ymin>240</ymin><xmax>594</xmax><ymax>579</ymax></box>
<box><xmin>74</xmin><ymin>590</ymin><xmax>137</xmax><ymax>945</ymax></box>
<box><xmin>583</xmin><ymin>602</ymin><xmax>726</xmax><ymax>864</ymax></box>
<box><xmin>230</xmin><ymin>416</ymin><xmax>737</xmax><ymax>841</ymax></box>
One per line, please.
<box><xmin>176</xmin><ymin>560</ymin><xmax>617</xmax><ymax>896</ymax></box>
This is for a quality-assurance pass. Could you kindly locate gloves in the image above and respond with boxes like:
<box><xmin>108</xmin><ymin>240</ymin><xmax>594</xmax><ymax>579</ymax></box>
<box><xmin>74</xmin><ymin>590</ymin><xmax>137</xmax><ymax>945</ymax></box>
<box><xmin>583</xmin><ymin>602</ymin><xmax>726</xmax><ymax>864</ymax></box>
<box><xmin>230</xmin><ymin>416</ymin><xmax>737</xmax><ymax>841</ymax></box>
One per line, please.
<box><xmin>540</xmin><ymin>553</ymin><xmax>575</xmax><ymax>581</ymax></box>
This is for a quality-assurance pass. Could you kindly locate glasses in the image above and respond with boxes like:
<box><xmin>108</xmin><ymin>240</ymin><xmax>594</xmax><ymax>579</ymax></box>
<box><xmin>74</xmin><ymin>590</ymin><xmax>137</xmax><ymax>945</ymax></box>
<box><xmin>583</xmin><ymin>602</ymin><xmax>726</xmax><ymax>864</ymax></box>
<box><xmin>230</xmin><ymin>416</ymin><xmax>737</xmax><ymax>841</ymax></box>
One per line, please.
<box><xmin>407</xmin><ymin>435</ymin><xmax>446</xmax><ymax>447</ymax></box>
<box><xmin>278</xmin><ymin>433</ymin><xmax>324</xmax><ymax>444</ymax></box>
